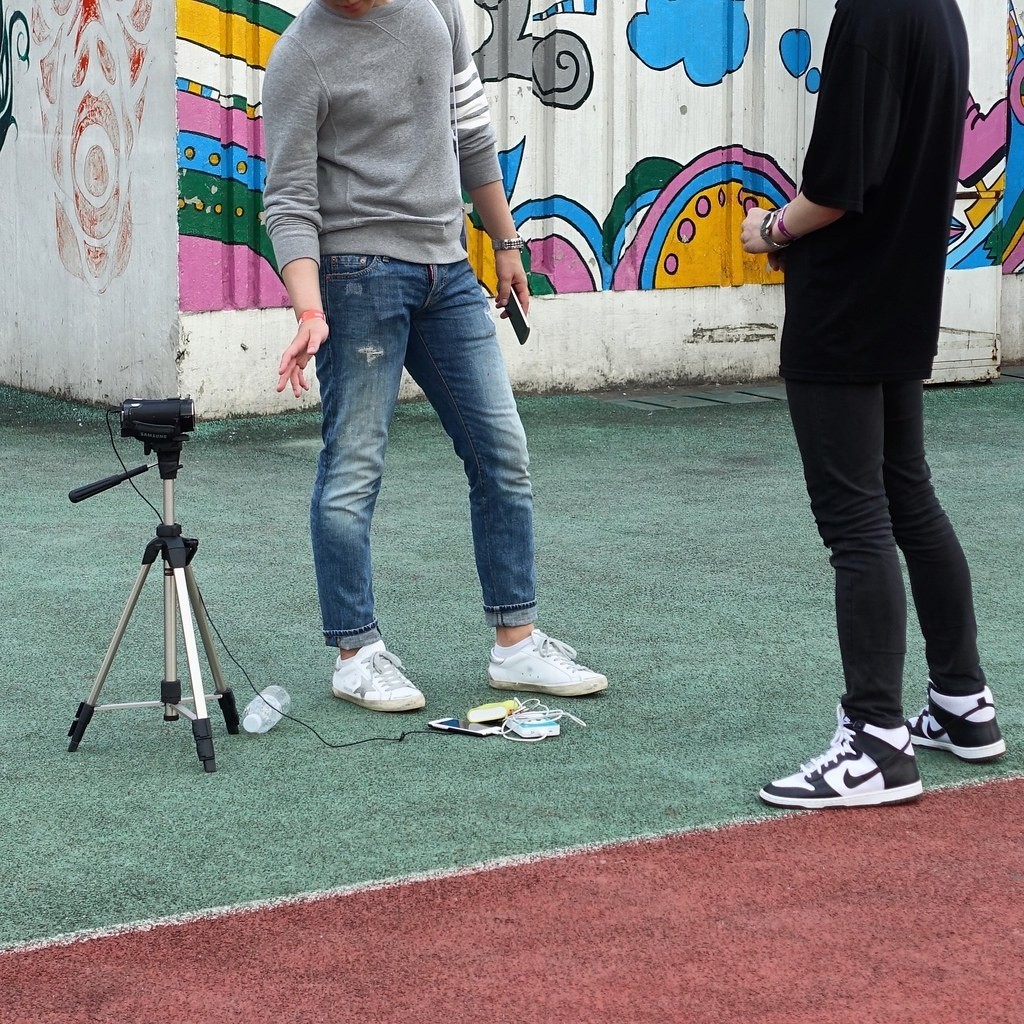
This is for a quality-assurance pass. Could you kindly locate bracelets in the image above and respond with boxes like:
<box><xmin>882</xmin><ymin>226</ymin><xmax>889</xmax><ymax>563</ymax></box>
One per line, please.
<box><xmin>778</xmin><ymin>203</ymin><xmax>798</xmax><ymax>241</ymax></box>
<box><xmin>298</xmin><ymin>310</ymin><xmax>326</xmax><ymax>326</ymax></box>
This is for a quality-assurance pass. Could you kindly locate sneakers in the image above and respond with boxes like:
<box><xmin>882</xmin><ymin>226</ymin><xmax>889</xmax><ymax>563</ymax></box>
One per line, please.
<box><xmin>904</xmin><ymin>682</ymin><xmax>1007</xmax><ymax>761</ymax></box>
<box><xmin>332</xmin><ymin>640</ymin><xmax>425</xmax><ymax>711</ymax></box>
<box><xmin>488</xmin><ymin>629</ymin><xmax>608</xmax><ymax>696</ymax></box>
<box><xmin>759</xmin><ymin>703</ymin><xmax>924</xmax><ymax>809</ymax></box>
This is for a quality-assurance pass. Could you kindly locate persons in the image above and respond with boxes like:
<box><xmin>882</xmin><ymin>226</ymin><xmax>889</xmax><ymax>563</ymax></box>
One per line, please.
<box><xmin>261</xmin><ymin>0</ymin><xmax>609</xmax><ymax>711</ymax></box>
<box><xmin>740</xmin><ymin>0</ymin><xmax>1007</xmax><ymax>809</ymax></box>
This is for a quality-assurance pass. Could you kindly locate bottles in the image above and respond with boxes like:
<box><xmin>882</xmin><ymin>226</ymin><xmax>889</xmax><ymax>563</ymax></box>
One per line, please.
<box><xmin>239</xmin><ymin>684</ymin><xmax>291</xmax><ymax>733</ymax></box>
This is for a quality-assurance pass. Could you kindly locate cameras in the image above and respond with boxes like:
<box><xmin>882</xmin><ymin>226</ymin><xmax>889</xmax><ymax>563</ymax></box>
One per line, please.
<box><xmin>118</xmin><ymin>398</ymin><xmax>194</xmax><ymax>441</ymax></box>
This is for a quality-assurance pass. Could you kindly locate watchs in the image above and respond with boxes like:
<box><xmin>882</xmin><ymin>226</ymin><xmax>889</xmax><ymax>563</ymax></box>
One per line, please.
<box><xmin>760</xmin><ymin>209</ymin><xmax>791</xmax><ymax>250</ymax></box>
<box><xmin>492</xmin><ymin>231</ymin><xmax>524</xmax><ymax>252</ymax></box>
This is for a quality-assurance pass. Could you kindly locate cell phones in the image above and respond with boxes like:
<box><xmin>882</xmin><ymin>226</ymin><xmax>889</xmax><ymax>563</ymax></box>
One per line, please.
<box><xmin>496</xmin><ymin>282</ymin><xmax>530</xmax><ymax>346</ymax></box>
<box><xmin>429</xmin><ymin>718</ymin><xmax>500</xmax><ymax>736</ymax></box>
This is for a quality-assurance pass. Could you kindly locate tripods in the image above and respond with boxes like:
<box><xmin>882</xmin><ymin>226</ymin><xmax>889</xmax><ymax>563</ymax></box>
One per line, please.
<box><xmin>62</xmin><ymin>441</ymin><xmax>241</xmax><ymax>771</ymax></box>
